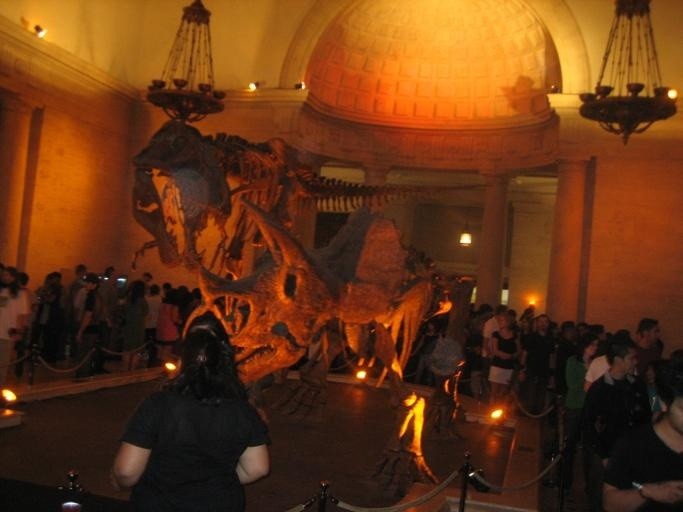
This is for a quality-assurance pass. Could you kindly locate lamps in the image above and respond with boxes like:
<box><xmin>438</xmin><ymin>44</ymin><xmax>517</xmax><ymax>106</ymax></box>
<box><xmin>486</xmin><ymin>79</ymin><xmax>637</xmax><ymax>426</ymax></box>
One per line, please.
<box><xmin>576</xmin><ymin>1</ymin><xmax>679</xmax><ymax>148</ymax></box>
<box><xmin>144</xmin><ymin>0</ymin><xmax>228</xmax><ymax>126</ymax></box>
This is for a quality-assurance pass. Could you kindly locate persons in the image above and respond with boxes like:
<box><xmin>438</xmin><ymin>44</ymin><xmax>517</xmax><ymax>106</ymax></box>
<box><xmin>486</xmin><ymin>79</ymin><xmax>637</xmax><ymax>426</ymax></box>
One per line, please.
<box><xmin>0</xmin><ymin>263</ymin><xmax>233</xmax><ymax>390</ymax></box>
<box><xmin>402</xmin><ymin>299</ymin><xmax>683</xmax><ymax>510</ymax></box>
<box><xmin>110</xmin><ymin>324</ymin><xmax>273</xmax><ymax>512</ymax></box>
